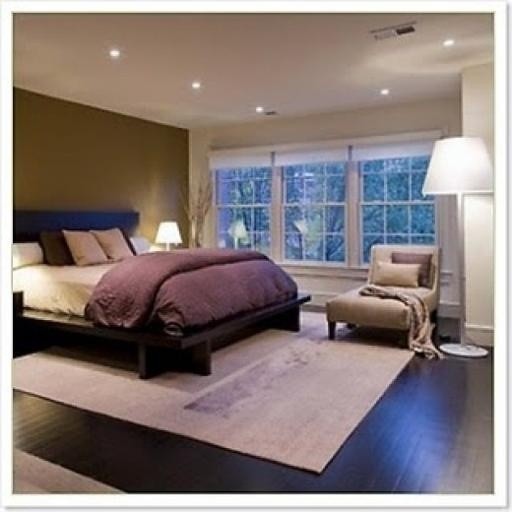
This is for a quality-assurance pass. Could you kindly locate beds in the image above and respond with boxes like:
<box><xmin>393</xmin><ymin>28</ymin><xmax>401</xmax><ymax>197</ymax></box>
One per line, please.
<box><xmin>13</xmin><ymin>211</ymin><xmax>310</xmax><ymax>380</ymax></box>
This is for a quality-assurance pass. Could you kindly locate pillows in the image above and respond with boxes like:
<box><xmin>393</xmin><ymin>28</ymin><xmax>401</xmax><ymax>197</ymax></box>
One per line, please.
<box><xmin>392</xmin><ymin>251</ymin><xmax>433</xmax><ymax>287</ymax></box>
<box><xmin>13</xmin><ymin>226</ymin><xmax>149</xmax><ymax>269</ymax></box>
<box><xmin>372</xmin><ymin>259</ymin><xmax>421</xmax><ymax>287</ymax></box>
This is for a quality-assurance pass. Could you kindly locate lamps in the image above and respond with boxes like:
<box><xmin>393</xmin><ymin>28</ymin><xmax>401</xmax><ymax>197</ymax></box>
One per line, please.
<box><xmin>154</xmin><ymin>220</ymin><xmax>183</xmax><ymax>251</ymax></box>
<box><xmin>422</xmin><ymin>137</ymin><xmax>496</xmax><ymax>357</ymax></box>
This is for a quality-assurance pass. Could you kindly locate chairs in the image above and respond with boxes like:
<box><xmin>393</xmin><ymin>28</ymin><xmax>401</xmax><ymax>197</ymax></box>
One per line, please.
<box><xmin>326</xmin><ymin>244</ymin><xmax>441</xmax><ymax>340</ymax></box>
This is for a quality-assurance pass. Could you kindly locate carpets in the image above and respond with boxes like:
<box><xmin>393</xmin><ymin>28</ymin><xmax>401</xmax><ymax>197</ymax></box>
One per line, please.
<box><xmin>13</xmin><ymin>311</ymin><xmax>416</xmax><ymax>473</ymax></box>
